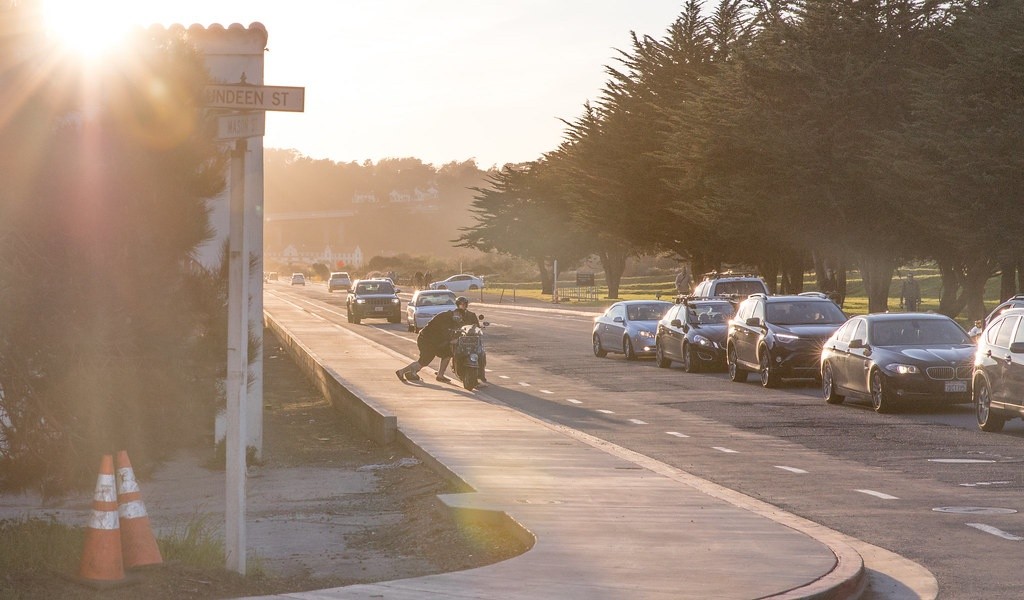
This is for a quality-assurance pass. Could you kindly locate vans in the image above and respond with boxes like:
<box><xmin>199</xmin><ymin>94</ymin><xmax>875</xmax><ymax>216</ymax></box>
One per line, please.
<box><xmin>692</xmin><ymin>268</ymin><xmax>771</xmax><ymax>297</ymax></box>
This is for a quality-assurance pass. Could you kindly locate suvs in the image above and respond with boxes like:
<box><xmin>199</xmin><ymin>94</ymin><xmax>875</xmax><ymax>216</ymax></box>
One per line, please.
<box><xmin>654</xmin><ymin>296</ymin><xmax>738</xmax><ymax>374</ymax></box>
<box><xmin>346</xmin><ymin>278</ymin><xmax>402</xmax><ymax>324</ymax></box>
<box><xmin>727</xmin><ymin>291</ymin><xmax>850</xmax><ymax>388</ymax></box>
<box><xmin>967</xmin><ymin>294</ymin><xmax>1024</xmax><ymax>433</ymax></box>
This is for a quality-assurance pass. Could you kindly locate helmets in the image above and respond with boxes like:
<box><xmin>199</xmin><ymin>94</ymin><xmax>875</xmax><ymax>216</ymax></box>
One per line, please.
<box><xmin>455</xmin><ymin>296</ymin><xmax>469</xmax><ymax>308</ymax></box>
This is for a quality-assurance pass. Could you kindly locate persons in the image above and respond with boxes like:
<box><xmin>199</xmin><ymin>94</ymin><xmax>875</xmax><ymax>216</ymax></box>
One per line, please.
<box><xmin>675</xmin><ymin>266</ymin><xmax>694</xmax><ymax>294</ymax></box>
<box><xmin>434</xmin><ymin>296</ymin><xmax>488</xmax><ymax>383</ymax></box>
<box><xmin>901</xmin><ymin>272</ymin><xmax>920</xmax><ymax>310</ymax></box>
<box><xmin>820</xmin><ymin>267</ymin><xmax>838</xmax><ymax>302</ymax></box>
<box><xmin>424</xmin><ymin>270</ymin><xmax>433</xmax><ymax>287</ymax></box>
<box><xmin>414</xmin><ymin>269</ymin><xmax>424</xmax><ymax>288</ymax></box>
<box><xmin>396</xmin><ymin>308</ymin><xmax>464</xmax><ymax>381</ymax></box>
<box><xmin>807</xmin><ymin>307</ymin><xmax>823</xmax><ymax>320</ymax></box>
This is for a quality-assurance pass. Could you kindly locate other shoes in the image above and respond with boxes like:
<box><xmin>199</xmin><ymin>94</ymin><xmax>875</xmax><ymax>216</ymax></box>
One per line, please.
<box><xmin>481</xmin><ymin>377</ymin><xmax>487</xmax><ymax>383</ymax></box>
<box><xmin>396</xmin><ymin>370</ymin><xmax>406</xmax><ymax>382</ymax></box>
<box><xmin>435</xmin><ymin>370</ymin><xmax>439</xmax><ymax>374</ymax></box>
<box><xmin>435</xmin><ymin>375</ymin><xmax>451</xmax><ymax>382</ymax></box>
<box><xmin>405</xmin><ymin>371</ymin><xmax>420</xmax><ymax>381</ymax></box>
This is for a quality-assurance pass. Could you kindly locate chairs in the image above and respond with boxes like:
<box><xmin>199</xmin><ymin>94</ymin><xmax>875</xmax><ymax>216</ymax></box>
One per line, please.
<box><xmin>713</xmin><ymin>313</ymin><xmax>724</xmax><ymax>322</ymax></box>
<box><xmin>423</xmin><ymin>298</ymin><xmax>432</xmax><ymax>304</ymax></box>
<box><xmin>419</xmin><ymin>297</ymin><xmax>426</xmax><ymax>302</ymax></box>
<box><xmin>360</xmin><ymin>285</ymin><xmax>366</xmax><ymax>292</ymax></box>
<box><xmin>698</xmin><ymin>312</ymin><xmax>710</xmax><ymax>324</ymax></box>
<box><xmin>638</xmin><ymin>307</ymin><xmax>651</xmax><ymax>319</ymax></box>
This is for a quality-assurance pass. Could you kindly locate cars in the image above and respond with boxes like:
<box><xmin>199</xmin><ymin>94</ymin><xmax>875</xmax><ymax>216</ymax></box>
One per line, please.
<box><xmin>820</xmin><ymin>309</ymin><xmax>977</xmax><ymax>413</ymax></box>
<box><xmin>429</xmin><ymin>274</ymin><xmax>486</xmax><ymax>292</ymax></box>
<box><xmin>373</xmin><ymin>277</ymin><xmax>396</xmax><ymax>289</ymax></box>
<box><xmin>591</xmin><ymin>300</ymin><xmax>675</xmax><ymax>361</ymax></box>
<box><xmin>406</xmin><ymin>287</ymin><xmax>459</xmax><ymax>331</ymax></box>
<box><xmin>326</xmin><ymin>272</ymin><xmax>352</xmax><ymax>292</ymax></box>
<box><xmin>291</xmin><ymin>272</ymin><xmax>306</xmax><ymax>285</ymax></box>
<box><xmin>269</xmin><ymin>271</ymin><xmax>280</xmax><ymax>279</ymax></box>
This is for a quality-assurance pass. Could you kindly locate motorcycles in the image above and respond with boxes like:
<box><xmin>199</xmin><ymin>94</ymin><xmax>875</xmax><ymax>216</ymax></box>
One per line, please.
<box><xmin>444</xmin><ymin>315</ymin><xmax>490</xmax><ymax>390</ymax></box>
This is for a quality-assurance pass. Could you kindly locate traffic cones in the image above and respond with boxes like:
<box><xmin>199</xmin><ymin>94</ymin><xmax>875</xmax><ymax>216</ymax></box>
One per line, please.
<box><xmin>76</xmin><ymin>449</ymin><xmax>144</xmax><ymax>589</ymax></box>
<box><xmin>116</xmin><ymin>449</ymin><xmax>169</xmax><ymax>571</ymax></box>
